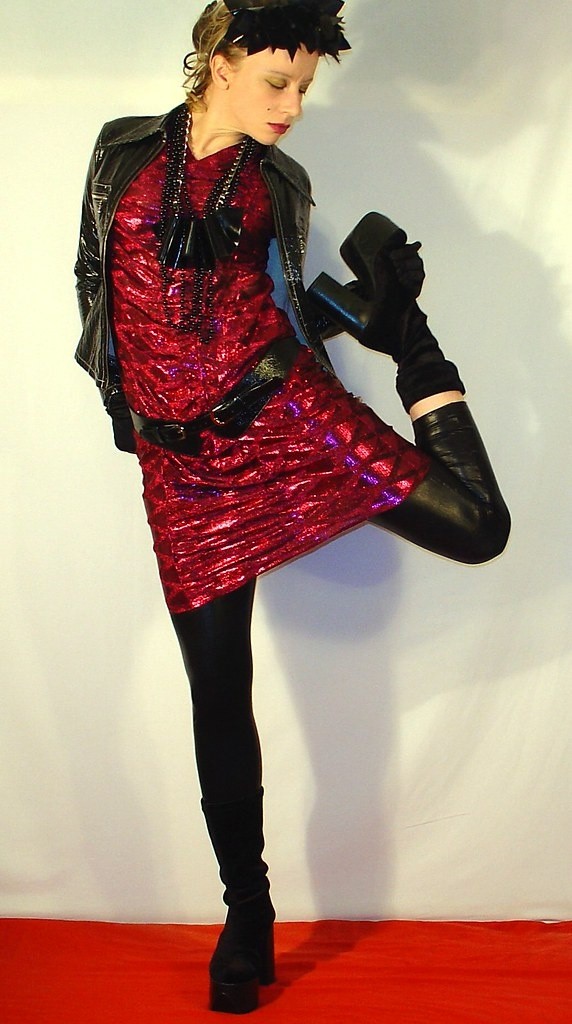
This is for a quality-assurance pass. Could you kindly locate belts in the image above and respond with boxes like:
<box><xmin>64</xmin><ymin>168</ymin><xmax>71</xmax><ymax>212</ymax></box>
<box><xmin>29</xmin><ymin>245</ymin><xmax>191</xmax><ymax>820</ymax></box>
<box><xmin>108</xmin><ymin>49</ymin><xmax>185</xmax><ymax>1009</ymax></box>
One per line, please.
<box><xmin>127</xmin><ymin>337</ymin><xmax>299</xmax><ymax>456</ymax></box>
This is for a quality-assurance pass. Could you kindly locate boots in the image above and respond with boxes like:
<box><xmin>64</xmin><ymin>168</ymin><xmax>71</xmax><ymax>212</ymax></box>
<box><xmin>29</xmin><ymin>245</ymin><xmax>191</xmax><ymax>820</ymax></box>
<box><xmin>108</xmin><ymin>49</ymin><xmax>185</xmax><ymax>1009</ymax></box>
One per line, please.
<box><xmin>307</xmin><ymin>211</ymin><xmax>464</xmax><ymax>412</ymax></box>
<box><xmin>199</xmin><ymin>789</ymin><xmax>277</xmax><ymax>1015</ymax></box>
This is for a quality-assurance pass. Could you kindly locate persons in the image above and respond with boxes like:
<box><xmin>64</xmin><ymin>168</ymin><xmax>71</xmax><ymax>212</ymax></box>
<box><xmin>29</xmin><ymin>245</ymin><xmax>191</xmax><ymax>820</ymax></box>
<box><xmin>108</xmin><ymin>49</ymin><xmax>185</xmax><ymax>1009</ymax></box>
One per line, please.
<box><xmin>73</xmin><ymin>0</ymin><xmax>510</xmax><ymax>1013</ymax></box>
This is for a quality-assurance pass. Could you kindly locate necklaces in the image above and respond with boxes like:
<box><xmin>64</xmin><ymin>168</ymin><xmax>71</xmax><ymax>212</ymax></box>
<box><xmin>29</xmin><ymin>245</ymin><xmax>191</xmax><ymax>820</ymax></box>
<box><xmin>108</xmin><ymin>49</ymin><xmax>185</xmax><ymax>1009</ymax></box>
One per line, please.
<box><xmin>157</xmin><ymin>112</ymin><xmax>257</xmax><ymax>342</ymax></box>
